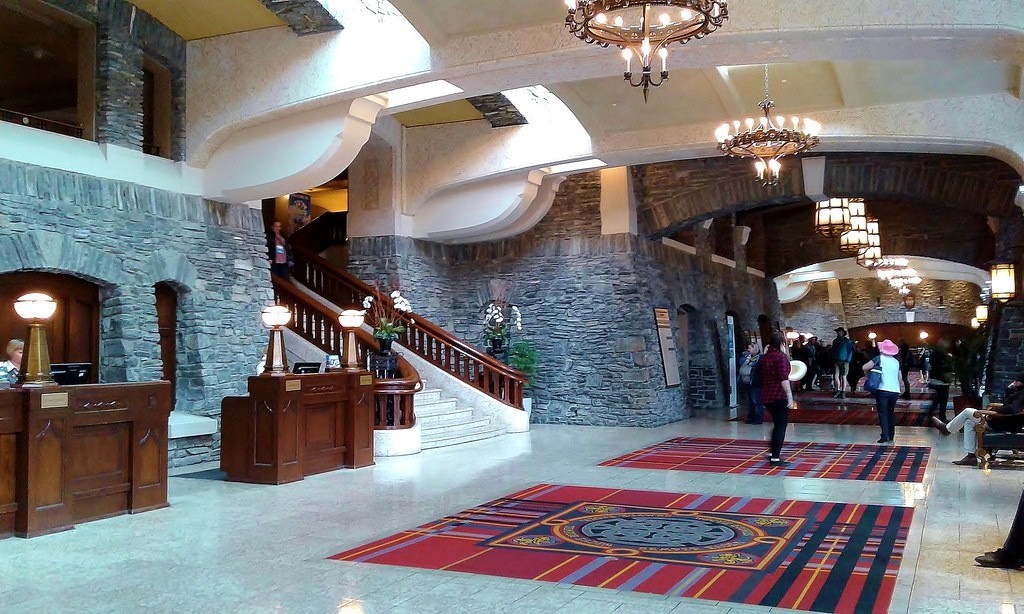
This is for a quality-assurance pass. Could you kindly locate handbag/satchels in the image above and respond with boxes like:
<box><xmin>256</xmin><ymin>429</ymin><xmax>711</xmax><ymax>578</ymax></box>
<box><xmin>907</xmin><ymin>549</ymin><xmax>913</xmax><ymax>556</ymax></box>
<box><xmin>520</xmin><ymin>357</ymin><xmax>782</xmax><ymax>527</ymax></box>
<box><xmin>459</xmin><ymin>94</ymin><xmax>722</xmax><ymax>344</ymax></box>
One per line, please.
<box><xmin>864</xmin><ymin>355</ymin><xmax>882</xmax><ymax>392</ymax></box>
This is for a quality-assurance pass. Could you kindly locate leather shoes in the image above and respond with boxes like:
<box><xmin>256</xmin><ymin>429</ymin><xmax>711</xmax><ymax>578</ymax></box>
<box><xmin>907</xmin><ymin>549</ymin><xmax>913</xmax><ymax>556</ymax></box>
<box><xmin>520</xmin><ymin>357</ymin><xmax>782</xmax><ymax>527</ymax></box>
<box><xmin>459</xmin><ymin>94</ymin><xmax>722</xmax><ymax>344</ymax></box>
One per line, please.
<box><xmin>770</xmin><ymin>459</ymin><xmax>791</xmax><ymax>466</ymax></box>
<box><xmin>954</xmin><ymin>454</ymin><xmax>978</xmax><ymax>466</ymax></box>
<box><xmin>932</xmin><ymin>415</ymin><xmax>950</xmax><ymax>437</ymax></box>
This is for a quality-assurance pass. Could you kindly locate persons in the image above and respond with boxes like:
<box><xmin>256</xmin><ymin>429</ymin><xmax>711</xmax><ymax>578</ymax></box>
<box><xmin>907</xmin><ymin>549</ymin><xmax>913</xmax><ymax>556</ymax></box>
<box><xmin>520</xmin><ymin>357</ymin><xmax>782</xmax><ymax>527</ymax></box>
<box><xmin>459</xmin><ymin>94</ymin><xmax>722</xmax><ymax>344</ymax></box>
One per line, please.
<box><xmin>740</xmin><ymin>327</ymin><xmax>970</xmax><ymax>443</ymax></box>
<box><xmin>0</xmin><ymin>338</ymin><xmax>24</xmax><ymax>387</ymax></box>
<box><xmin>930</xmin><ymin>373</ymin><xmax>1024</xmax><ymax>466</ymax></box>
<box><xmin>760</xmin><ymin>331</ymin><xmax>797</xmax><ymax>466</ymax></box>
<box><xmin>975</xmin><ymin>490</ymin><xmax>1024</xmax><ymax>568</ymax></box>
<box><xmin>266</xmin><ymin>222</ymin><xmax>290</xmax><ymax>305</ymax></box>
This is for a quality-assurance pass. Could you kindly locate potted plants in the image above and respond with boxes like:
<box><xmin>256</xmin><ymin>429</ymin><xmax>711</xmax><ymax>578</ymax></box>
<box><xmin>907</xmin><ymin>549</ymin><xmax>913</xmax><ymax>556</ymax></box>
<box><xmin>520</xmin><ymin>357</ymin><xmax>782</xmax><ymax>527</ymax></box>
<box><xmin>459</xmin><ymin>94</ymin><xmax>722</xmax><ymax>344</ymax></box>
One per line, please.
<box><xmin>509</xmin><ymin>343</ymin><xmax>539</xmax><ymax>417</ymax></box>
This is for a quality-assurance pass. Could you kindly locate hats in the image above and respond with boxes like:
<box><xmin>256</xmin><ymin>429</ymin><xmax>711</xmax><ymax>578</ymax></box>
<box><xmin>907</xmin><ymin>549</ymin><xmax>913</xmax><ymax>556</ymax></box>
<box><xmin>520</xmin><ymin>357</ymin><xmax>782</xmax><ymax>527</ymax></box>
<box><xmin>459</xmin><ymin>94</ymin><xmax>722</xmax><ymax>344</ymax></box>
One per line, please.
<box><xmin>806</xmin><ymin>333</ymin><xmax>818</xmax><ymax>341</ymax></box>
<box><xmin>835</xmin><ymin>327</ymin><xmax>847</xmax><ymax>336</ymax></box>
<box><xmin>877</xmin><ymin>339</ymin><xmax>899</xmax><ymax>355</ymax></box>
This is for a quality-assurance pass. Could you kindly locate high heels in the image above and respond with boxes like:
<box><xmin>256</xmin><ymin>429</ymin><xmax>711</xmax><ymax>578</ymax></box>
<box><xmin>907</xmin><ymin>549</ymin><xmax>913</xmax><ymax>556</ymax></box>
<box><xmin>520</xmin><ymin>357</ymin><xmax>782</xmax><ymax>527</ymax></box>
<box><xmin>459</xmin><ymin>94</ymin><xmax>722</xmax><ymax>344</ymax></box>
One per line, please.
<box><xmin>877</xmin><ymin>436</ymin><xmax>893</xmax><ymax>443</ymax></box>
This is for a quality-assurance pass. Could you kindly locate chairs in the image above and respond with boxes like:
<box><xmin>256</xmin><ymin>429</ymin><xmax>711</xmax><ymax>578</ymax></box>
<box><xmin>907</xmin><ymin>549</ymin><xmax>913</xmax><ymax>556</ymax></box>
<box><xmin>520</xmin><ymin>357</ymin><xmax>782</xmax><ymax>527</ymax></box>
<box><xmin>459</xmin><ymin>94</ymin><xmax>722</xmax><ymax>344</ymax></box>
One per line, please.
<box><xmin>973</xmin><ymin>403</ymin><xmax>1024</xmax><ymax>470</ymax></box>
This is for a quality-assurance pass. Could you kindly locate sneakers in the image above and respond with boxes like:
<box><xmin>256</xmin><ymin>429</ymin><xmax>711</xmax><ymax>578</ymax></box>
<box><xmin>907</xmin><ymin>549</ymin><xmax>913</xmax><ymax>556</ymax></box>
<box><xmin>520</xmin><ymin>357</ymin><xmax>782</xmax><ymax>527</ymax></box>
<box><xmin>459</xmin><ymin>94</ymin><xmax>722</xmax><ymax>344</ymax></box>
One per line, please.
<box><xmin>975</xmin><ymin>547</ymin><xmax>1022</xmax><ymax>569</ymax></box>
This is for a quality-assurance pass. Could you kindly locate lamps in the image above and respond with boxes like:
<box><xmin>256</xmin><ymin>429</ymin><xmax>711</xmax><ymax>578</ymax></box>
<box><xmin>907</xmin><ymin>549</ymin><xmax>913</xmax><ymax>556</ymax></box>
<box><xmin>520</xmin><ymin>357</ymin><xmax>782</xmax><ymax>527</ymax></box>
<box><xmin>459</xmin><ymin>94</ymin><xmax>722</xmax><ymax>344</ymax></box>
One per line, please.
<box><xmin>814</xmin><ymin>198</ymin><xmax>922</xmax><ymax>309</ymax></box>
<box><xmin>972</xmin><ymin>257</ymin><xmax>1024</xmax><ymax>333</ymax></box>
<box><xmin>564</xmin><ymin>0</ymin><xmax>728</xmax><ymax>104</ymax></box>
<box><xmin>716</xmin><ymin>64</ymin><xmax>820</xmax><ymax>188</ymax></box>
<box><xmin>12</xmin><ymin>290</ymin><xmax>59</xmax><ymax>388</ymax></box>
<box><xmin>261</xmin><ymin>305</ymin><xmax>292</xmax><ymax>375</ymax></box>
<box><xmin>337</xmin><ymin>309</ymin><xmax>364</xmax><ymax>370</ymax></box>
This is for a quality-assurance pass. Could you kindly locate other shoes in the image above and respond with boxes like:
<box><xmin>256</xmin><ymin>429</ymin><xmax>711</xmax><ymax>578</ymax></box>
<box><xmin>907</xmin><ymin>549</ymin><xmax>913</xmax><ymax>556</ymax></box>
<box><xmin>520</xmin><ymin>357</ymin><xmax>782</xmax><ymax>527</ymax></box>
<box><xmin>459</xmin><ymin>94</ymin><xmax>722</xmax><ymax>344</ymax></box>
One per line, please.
<box><xmin>833</xmin><ymin>391</ymin><xmax>841</xmax><ymax>398</ymax></box>
<box><xmin>899</xmin><ymin>392</ymin><xmax>910</xmax><ymax>399</ymax></box>
<box><xmin>851</xmin><ymin>386</ymin><xmax>856</xmax><ymax>392</ymax></box>
<box><xmin>841</xmin><ymin>392</ymin><xmax>845</xmax><ymax>399</ymax></box>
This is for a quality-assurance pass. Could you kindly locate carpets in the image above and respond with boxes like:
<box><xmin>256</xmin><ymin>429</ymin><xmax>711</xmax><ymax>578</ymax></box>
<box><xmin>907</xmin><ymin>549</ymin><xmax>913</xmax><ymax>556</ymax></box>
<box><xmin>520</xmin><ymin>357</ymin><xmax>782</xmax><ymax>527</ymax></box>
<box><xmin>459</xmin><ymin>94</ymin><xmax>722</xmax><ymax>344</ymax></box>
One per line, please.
<box><xmin>596</xmin><ymin>437</ymin><xmax>931</xmax><ymax>484</ymax></box>
<box><xmin>326</xmin><ymin>484</ymin><xmax>916</xmax><ymax>614</ymax></box>
<box><xmin>728</xmin><ymin>371</ymin><xmax>963</xmax><ymax>427</ymax></box>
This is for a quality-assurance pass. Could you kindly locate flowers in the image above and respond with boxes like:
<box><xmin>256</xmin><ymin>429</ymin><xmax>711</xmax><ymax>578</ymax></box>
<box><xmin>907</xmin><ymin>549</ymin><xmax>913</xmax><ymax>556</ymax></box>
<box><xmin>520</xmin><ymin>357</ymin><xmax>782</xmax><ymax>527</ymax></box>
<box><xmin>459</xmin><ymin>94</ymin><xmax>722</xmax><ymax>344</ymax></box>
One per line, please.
<box><xmin>363</xmin><ymin>281</ymin><xmax>415</xmax><ymax>342</ymax></box>
<box><xmin>483</xmin><ymin>303</ymin><xmax>522</xmax><ymax>346</ymax></box>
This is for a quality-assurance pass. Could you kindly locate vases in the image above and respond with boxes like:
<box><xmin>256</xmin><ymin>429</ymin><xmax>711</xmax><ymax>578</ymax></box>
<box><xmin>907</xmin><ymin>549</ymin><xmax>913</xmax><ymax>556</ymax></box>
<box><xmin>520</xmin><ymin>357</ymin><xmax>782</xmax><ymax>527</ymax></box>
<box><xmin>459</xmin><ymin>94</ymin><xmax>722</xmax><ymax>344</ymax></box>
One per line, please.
<box><xmin>487</xmin><ymin>339</ymin><xmax>508</xmax><ymax>366</ymax></box>
<box><xmin>372</xmin><ymin>339</ymin><xmax>398</xmax><ymax>380</ymax></box>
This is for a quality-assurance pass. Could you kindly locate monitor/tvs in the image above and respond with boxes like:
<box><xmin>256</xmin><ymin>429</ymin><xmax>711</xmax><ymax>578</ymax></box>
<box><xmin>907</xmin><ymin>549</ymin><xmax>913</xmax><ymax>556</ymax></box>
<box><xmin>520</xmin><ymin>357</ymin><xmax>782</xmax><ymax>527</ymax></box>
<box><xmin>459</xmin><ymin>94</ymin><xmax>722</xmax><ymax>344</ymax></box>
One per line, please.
<box><xmin>50</xmin><ymin>363</ymin><xmax>92</xmax><ymax>385</ymax></box>
<box><xmin>293</xmin><ymin>362</ymin><xmax>321</xmax><ymax>374</ymax></box>
